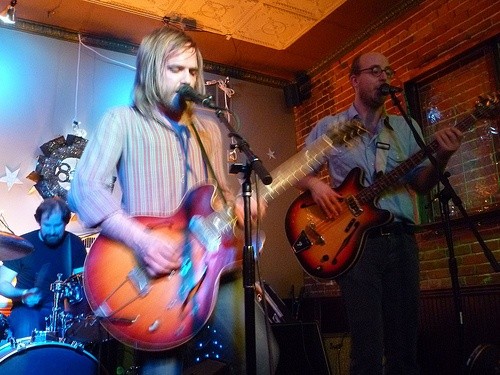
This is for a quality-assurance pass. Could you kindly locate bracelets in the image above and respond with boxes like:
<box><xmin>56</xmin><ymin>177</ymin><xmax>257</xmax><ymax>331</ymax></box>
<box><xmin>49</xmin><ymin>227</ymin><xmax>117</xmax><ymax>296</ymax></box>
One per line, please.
<box><xmin>22</xmin><ymin>289</ymin><xmax>28</xmax><ymax>304</ymax></box>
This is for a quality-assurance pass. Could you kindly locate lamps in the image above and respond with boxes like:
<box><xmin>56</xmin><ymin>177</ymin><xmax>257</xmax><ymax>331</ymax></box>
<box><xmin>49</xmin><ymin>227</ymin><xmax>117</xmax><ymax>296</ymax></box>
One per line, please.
<box><xmin>0</xmin><ymin>0</ymin><xmax>17</xmax><ymax>24</ymax></box>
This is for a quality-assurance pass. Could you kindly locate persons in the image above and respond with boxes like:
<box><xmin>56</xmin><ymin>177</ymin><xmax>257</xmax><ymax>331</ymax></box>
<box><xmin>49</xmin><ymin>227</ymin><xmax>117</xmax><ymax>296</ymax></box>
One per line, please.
<box><xmin>68</xmin><ymin>27</ymin><xmax>279</xmax><ymax>375</ymax></box>
<box><xmin>0</xmin><ymin>198</ymin><xmax>88</xmax><ymax>340</ymax></box>
<box><xmin>293</xmin><ymin>52</ymin><xmax>464</xmax><ymax>375</ymax></box>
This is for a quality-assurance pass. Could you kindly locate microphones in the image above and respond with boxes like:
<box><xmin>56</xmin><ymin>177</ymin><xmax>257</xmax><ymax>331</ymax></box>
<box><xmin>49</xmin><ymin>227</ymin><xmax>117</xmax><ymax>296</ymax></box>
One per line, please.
<box><xmin>379</xmin><ymin>83</ymin><xmax>403</xmax><ymax>96</ymax></box>
<box><xmin>178</xmin><ymin>85</ymin><xmax>217</xmax><ymax>109</ymax></box>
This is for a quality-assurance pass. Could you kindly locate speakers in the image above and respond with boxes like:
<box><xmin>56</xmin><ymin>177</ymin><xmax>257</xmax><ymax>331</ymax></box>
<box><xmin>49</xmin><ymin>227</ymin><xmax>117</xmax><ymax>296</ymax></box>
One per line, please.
<box><xmin>283</xmin><ymin>84</ymin><xmax>301</xmax><ymax>108</ymax></box>
<box><xmin>261</xmin><ymin>320</ymin><xmax>353</xmax><ymax>375</ymax></box>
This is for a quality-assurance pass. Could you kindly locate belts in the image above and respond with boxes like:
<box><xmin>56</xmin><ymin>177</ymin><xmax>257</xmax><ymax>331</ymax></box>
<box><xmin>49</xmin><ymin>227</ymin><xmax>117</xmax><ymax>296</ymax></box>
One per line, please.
<box><xmin>366</xmin><ymin>223</ymin><xmax>413</xmax><ymax>235</ymax></box>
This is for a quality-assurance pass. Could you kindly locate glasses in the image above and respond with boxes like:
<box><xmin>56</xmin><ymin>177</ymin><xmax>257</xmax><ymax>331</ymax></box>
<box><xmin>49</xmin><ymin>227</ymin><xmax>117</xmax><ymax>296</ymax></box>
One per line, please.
<box><xmin>357</xmin><ymin>65</ymin><xmax>395</xmax><ymax>79</ymax></box>
